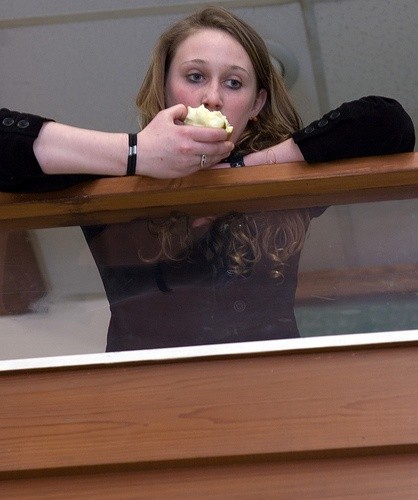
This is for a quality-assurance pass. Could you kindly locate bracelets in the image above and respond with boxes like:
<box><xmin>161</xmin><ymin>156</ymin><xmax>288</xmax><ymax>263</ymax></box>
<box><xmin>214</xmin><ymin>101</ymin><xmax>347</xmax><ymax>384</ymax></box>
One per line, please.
<box><xmin>229</xmin><ymin>154</ymin><xmax>245</xmax><ymax>170</ymax></box>
<box><xmin>127</xmin><ymin>133</ymin><xmax>138</xmax><ymax>176</ymax></box>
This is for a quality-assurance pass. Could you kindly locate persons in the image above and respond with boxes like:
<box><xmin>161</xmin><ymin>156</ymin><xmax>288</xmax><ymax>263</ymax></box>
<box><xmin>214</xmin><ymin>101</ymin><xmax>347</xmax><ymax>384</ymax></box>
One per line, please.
<box><xmin>0</xmin><ymin>5</ymin><xmax>416</xmax><ymax>352</ymax></box>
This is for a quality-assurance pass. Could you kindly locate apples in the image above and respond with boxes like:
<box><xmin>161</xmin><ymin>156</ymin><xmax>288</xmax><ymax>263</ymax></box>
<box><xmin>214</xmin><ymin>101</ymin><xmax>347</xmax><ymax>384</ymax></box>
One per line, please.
<box><xmin>183</xmin><ymin>103</ymin><xmax>235</xmax><ymax>141</ymax></box>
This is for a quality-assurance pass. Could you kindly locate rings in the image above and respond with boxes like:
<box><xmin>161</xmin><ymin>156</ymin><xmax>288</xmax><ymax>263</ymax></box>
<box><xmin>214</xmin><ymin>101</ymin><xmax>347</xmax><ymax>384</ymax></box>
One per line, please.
<box><xmin>201</xmin><ymin>154</ymin><xmax>207</xmax><ymax>167</ymax></box>
